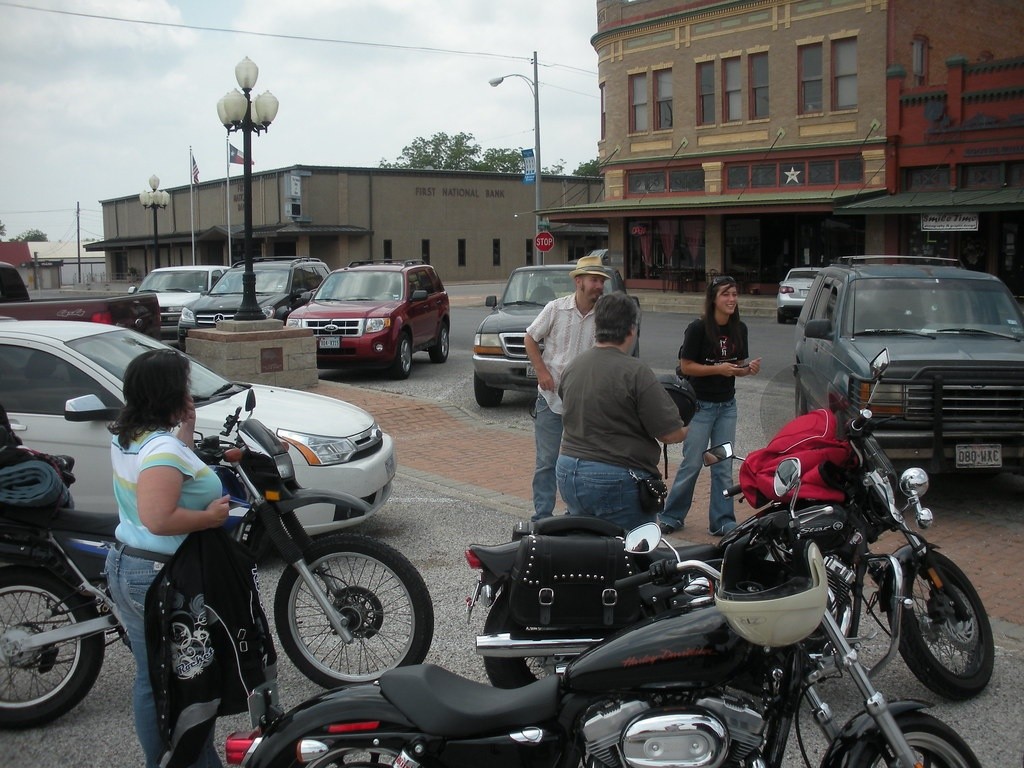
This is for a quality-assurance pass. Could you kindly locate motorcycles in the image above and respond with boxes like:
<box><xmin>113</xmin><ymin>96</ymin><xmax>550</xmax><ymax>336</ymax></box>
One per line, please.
<box><xmin>0</xmin><ymin>388</ymin><xmax>434</xmax><ymax>731</ymax></box>
<box><xmin>227</xmin><ymin>456</ymin><xmax>983</xmax><ymax>768</ymax></box>
<box><xmin>464</xmin><ymin>348</ymin><xmax>994</xmax><ymax>702</ymax></box>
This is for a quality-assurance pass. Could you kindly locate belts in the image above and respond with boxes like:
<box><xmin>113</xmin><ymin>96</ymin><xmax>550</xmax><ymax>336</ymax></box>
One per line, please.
<box><xmin>114</xmin><ymin>540</ymin><xmax>173</xmax><ymax>564</ymax></box>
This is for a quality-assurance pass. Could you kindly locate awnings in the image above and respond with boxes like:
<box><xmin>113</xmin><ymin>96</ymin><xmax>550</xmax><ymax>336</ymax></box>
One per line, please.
<box><xmin>532</xmin><ymin>187</ymin><xmax>1024</xmax><ymax>222</ymax></box>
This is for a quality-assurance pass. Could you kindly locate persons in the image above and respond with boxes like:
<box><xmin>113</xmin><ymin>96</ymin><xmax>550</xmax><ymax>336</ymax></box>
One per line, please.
<box><xmin>631</xmin><ymin>539</ymin><xmax>649</xmax><ymax>552</ymax></box>
<box><xmin>100</xmin><ymin>352</ymin><xmax>230</xmax><ymax>768</ymax></box>
<box><xmin>704</xmin><ymin>446</ymin><xmax>727</xmax><ymax>464</ymax></box>
<box><xmin>524</xmin><ymin>255</ymin><xmax>612</xmax><ymax>522</ymax></box>
<box><xmin>658</xmin><ymin>276</ymin><xmax>762</xmax><ymax>537</ymax></box>
<box><xmin>556</xmin><ymin>292</ymin><xmax>689</xmax><ymax>533</ymax></box>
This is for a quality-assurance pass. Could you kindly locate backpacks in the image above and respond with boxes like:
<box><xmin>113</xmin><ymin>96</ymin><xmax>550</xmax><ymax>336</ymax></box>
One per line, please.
<box><xmin>676</xmin><ymin>317</ymin><xmax>704</xmax><ymax>379</ymax></box>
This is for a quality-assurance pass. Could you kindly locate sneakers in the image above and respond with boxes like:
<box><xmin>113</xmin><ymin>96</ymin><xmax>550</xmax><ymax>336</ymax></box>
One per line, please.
<box><xmin>659</xmin><ymin>523</ymin><xmax>674</xmax><ymax>534</ymax></box>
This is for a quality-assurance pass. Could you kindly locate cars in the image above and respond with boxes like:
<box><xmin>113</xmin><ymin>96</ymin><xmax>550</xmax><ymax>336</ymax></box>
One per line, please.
<box><xmin>1</xmin><ymin>316</ymin><xmax>397</xmax><ymax>538</ymax></box>
<box><xmin>777</xmin><ymin>267</ymin><xmax>821</xmax><ymax>323</ymax></box>
<box><xmin>127</xmin><ymin>266</ymin><xmax>233</xmax><ymax>332</ymax></box>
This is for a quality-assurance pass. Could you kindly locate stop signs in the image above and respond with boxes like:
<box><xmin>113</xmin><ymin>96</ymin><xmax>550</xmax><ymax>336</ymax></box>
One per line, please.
<box><xmin>534</xmin><ymin>231</ymin><xmax>555</xmax><ymax>252</ymax></box>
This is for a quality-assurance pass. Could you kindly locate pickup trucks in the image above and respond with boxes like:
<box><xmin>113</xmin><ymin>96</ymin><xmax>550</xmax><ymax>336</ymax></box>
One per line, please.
<box><xmin>0</xmin><ymin>261</ymin><xmax>163</xmax><ymax>341</ymax></box>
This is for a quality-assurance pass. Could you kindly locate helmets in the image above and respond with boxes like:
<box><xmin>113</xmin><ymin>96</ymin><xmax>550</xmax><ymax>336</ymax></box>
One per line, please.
<box><xmin>715</xmin><ymin>532</ymin><xmax>828</xmax><ymax>654</ymax></box>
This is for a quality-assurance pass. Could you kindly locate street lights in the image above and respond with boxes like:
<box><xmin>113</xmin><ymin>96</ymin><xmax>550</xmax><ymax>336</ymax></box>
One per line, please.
<box><xmin>216</xmin><ymin>54</ymin><xmax>280</xmax><ymax>320</ymax></box>
<box><xmin>488</xmin><ymin>73</ymin><xmax>544</xmax><ymax>264</ymax></box>
<box><xmin>139</xmin><ymin>173</ymin><xmax>171</xmax><ymax>268</ymax></box>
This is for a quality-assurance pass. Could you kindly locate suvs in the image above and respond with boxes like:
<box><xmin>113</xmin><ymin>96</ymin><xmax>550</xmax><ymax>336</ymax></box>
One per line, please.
<box><xmin>473</xmin><ymin>264</ymin><xmax>642</xmax><ymax>407</ymax></box>
<box><xmin>568</xmin><ymin>249</ymin><xmax>609</xmax><ymax>266</ymax></box>
<box><xmin>791</xmin><ymin>253</ymin><xmax>1024</xmax><ymax>474</ymax></box>
<box><xmin>177</xmin><ymin>257</ymin><xmax>334</xmax><ymax>353</ymax></box>
<box><xmin>286</xmin><ymin>259</ymin><xmax>450</xmax><ymax>378</ymax></box>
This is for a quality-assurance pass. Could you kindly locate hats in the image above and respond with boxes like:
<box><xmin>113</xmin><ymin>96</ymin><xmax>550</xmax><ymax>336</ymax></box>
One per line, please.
<box><xmin>569</xmin><ymin>256</ymin><xmax>612</xmax><ymax>281</ymax></box>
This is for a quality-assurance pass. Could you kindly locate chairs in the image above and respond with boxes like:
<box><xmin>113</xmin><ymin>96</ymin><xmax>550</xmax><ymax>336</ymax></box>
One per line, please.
<box><xmin>528</xmin><ymin>286</ymin><xmax>556</xmax><ymax>303</ymax></box>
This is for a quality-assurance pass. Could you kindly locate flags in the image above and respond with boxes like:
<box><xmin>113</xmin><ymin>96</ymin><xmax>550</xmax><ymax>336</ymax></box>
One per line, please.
<box><xmin>229</xmin><ymin>143</ymin><xmax>255</xmax><ymax>165</ymax></box>
<box><xmin>192</xmin><ymin>154</ymin><xmax>199</xmax><ymax>186</ymax></box>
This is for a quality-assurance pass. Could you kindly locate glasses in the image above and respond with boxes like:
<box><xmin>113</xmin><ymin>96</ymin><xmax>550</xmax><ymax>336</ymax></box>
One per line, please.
<box><xmin>712</xmin><ymin>276</ymin><xmax>736</xmax><ymax>286</ymax></box>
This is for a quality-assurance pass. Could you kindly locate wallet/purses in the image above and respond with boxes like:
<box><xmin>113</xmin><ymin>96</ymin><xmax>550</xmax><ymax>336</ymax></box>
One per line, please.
<box><xmin>638</xmin><ymin>479</ymin><xmax>668</xmax><ymax>515</ymax></box>
